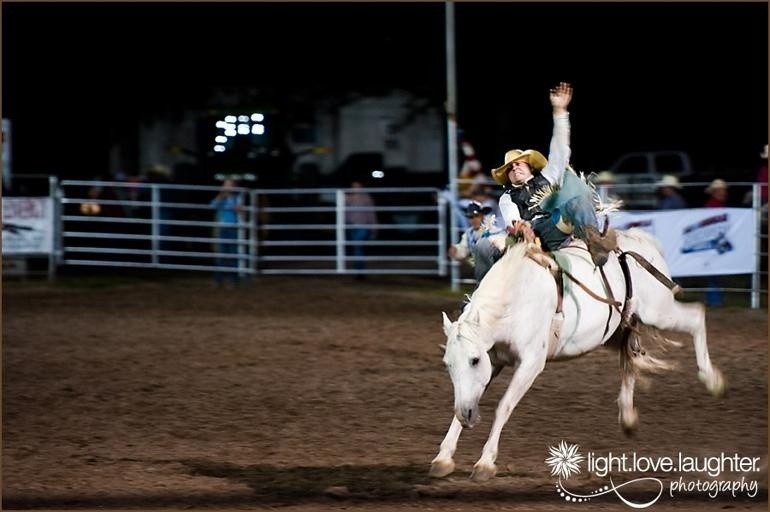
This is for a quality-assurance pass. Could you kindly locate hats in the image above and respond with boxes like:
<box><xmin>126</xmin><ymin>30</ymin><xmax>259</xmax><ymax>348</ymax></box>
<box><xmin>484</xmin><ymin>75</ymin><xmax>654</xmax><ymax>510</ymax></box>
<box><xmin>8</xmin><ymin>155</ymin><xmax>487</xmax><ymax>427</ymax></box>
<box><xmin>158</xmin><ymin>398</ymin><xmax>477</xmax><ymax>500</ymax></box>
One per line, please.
<box><xmin>657</xmin><ymin>174</ymin><xmax>682</xmax><ymax>189</ymax></box>
<box><xmin>462</xmin><ymin>203</ymin><xmax>491</xmax><ymax>218</ymax></box>
<box><xmin>491</xmin><ymin>147</ymin><xmax>547</xmax><ymax>184</ymax></box>
<box><xmin>761</xmin><ymin>144</ymin><xmax>769</xmax><ymax>157</ymax></box>
<box><xmin>593</xmin><ymin>171</ymin><xmax>618</xmax><ymax>183</ymax></box>
<box><xmin>706</xmin><ymin>178</ymin><xmax>728</xmax><ymax>193</ymax></box>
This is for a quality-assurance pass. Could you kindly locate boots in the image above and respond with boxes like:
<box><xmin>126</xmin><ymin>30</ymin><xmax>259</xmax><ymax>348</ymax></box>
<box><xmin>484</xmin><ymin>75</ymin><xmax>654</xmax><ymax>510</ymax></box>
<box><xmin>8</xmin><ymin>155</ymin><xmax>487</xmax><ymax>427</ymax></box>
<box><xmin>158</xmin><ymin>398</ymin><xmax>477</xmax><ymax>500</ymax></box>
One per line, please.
<box><xmin>581</xmin><ymin>225</ymin><xmax>617</xmax><ymax>267</ymax></box>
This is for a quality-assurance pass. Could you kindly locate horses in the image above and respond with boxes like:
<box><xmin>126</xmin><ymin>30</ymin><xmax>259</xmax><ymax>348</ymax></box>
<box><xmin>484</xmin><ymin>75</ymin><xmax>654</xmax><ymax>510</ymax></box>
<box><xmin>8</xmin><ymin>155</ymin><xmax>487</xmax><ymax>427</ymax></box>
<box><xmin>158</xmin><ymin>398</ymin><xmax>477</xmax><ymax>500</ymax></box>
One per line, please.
<box><xmin>427</xmin><ymin>228</ymin><xmax>730</xmax><ymax>485</ymax></box>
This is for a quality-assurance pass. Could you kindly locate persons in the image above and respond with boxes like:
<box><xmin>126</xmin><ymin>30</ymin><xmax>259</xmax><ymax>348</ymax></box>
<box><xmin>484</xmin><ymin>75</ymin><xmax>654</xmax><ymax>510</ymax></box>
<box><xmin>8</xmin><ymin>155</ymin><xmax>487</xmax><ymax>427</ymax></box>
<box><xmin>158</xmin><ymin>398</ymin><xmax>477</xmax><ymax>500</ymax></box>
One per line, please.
<box><xmin>758</xmin><ymin>143</ymin><xmax>768</xmax><ymax>204</ymax></box>
<box><xmin>449</xmin><ymin>199</ymin><xmax>510</xmax><ymax>287</ymax></box>
<box><xmin>589</xmin><ymin>170</ymin><xmax>625</xmax><ymax>211</ymax></box>
<box><xmin>490</xmin><ymin>81</ymin><xmax>618</xmax><ymax>267</ymax></box>
<box><xmin>209</xmin><ymin>179</ymin><xmax>244</xmax><ymax>287</ymax></box>
<box><xmin>655</xmin><ymin>176</ymin><xmax>690</xmax><ymax>300</ymax></box>
<box><xmin>343</xmin><ymin>181</ymin><xmax>378</xmax><ymax>282</ymax></box>
<box><xmin>702</xmin><ymin>178</ymin><xmax>728</xmax><ymax>307</ymax></box>
